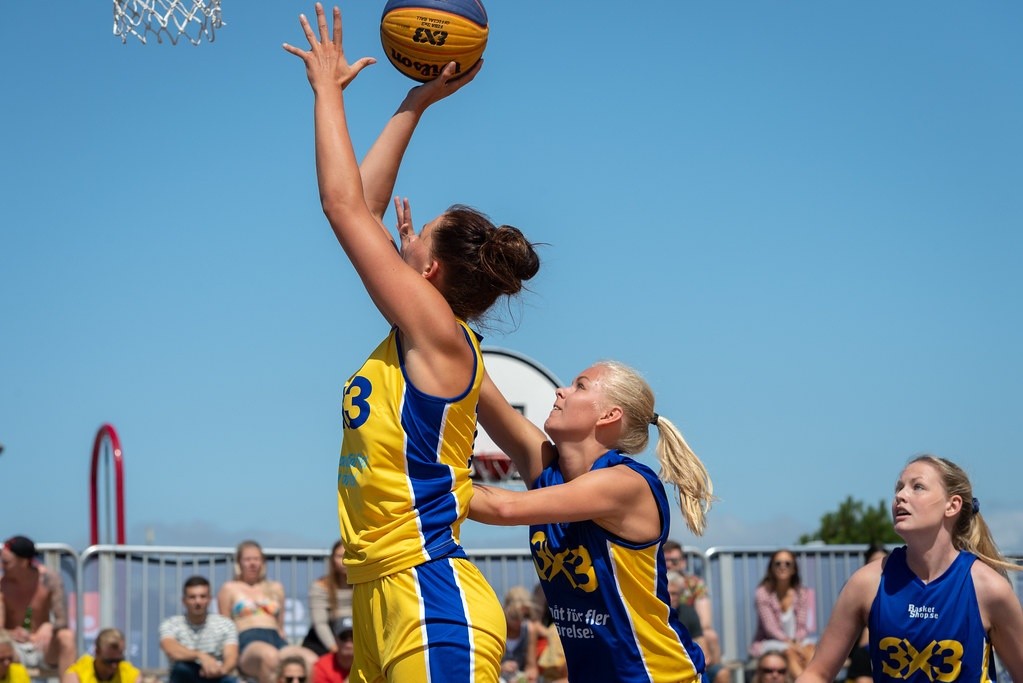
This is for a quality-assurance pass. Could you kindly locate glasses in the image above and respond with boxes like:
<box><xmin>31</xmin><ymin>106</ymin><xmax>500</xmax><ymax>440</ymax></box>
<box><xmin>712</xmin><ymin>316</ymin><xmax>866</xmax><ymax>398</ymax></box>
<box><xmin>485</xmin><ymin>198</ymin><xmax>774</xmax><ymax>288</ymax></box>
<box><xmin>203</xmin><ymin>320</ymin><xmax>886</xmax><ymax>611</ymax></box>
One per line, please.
<box><xmin>774</xmin><ymin>561</ymin><xmax>792</xmax><ymax>568</ymax></box>
<box><xmin>285</xmin><ymin>676</ymin><xmax>306</xmax><ymax>683</ymax></box>
<box><xmin>665</xmin><ymin>558</ymin><xmax>682</xmax><ymax>565</ymax></box>
<box><xmin>762</xmin><ymin>668</ymin><xmax>786</xmax><ymax>675</ymax></box>
<box><xmin>99</xmin><ymin>654</ymin><xmax>125</xmax><ymax>665</ymax></box>
<box><xmin>339</xmin><ymin>631</ymin><xmax>354</xmax><ymax>641</ymax></box>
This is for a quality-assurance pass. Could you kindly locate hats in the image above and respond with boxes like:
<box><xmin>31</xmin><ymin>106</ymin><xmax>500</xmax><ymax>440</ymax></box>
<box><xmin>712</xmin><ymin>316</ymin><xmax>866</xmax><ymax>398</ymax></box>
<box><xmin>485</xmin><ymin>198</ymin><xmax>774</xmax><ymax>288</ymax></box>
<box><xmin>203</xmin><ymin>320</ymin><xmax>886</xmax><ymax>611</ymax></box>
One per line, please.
<box><xmin>333</xmin><ymin>616</ymin><xmax>354</xmax><ymax>636</ymax></box>
<box><xmin>7</xmin><ymin>536</ymin><xmax>42</xmax><ymax>558</ymax></box>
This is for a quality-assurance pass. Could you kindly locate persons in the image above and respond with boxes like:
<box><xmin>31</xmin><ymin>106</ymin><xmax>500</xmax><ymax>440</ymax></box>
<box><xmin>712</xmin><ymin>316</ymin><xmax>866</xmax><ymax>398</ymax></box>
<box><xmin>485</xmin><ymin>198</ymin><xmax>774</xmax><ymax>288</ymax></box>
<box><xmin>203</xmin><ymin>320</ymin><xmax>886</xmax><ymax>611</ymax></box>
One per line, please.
<box><xmin>752</xmin><ymin>650</ymin><xmax>795</xmax><ymax>683</ymax></box>
<box><xmin>749</xmin><ymin>550</ymin><xmax>816</xmax><ymax>678</ymax></box>
<box><xmin>794</xmin><ymin>453</ymin><xmax>1023</xmax><ymax>683</ymax></box>
<box><xmin>498</xmin><ymin>584</ymin><xmax>569</xmax><ymax>683</ymax></box>
<box><xmin>0</xmin><ymin>536</ymin><xmax>143</xmax><ymax>683</ymax></box>
<box><xmin>159</xmin><ymin>540</ymin><xmax>354</xmax><ymax>683</ymax></box>
<box><xmin>663</xmin><ymin>540</ymin><xmax>731</xmax><ymax>683</ymax></box>
<box><xmin>282</xmin><ymin>2</ymin><xmax>539</xmax><ymax>683</ymax></box>
<box><xmin>394</xmin><ymin>196</ymin><xmax>715</xmax><ymax>683</ymax></box>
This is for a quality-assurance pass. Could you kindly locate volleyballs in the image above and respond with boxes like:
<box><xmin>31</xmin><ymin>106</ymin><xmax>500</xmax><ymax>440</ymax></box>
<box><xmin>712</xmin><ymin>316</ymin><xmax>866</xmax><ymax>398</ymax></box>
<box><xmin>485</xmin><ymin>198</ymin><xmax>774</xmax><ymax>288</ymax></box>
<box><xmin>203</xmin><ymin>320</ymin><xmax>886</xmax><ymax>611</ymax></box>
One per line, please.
<box><xmin>378</xmin><ymin>0</ymin><xmax>490</xmax><ymax>83</ymax></box>
